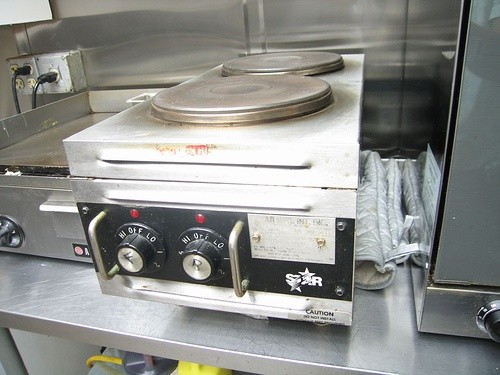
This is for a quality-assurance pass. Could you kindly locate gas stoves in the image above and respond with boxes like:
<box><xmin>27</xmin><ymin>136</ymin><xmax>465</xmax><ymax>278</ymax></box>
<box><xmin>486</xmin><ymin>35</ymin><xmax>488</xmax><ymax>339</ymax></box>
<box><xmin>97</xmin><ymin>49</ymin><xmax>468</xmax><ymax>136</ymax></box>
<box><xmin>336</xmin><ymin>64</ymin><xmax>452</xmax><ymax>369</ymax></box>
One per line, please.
<box><xmin>60</xmin><ymin>36</ymin><xmax>371</xmax><ymax>327</ymax></box>
